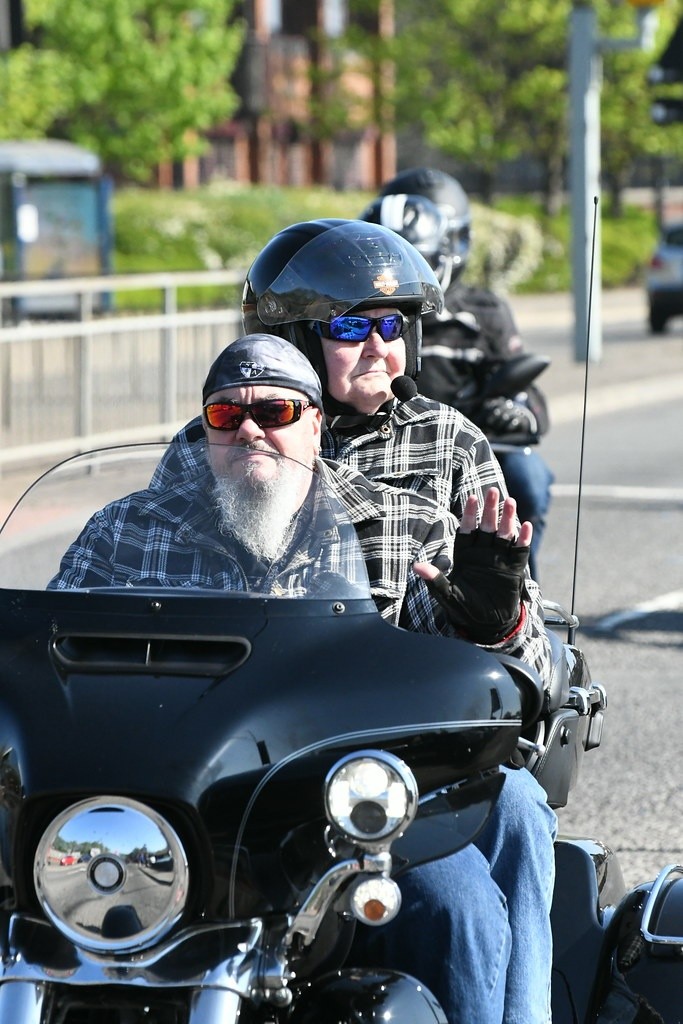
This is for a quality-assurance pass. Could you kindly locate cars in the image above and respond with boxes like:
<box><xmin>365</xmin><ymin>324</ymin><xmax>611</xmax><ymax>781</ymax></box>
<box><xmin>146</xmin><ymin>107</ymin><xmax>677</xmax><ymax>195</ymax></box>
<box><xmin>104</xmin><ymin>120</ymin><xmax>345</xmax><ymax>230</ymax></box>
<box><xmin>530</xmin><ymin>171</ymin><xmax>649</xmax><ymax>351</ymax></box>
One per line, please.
<box><xmin>644</xmin><ymin>221</ymin><xmax>683</xmax><ymax>336</ymax></box>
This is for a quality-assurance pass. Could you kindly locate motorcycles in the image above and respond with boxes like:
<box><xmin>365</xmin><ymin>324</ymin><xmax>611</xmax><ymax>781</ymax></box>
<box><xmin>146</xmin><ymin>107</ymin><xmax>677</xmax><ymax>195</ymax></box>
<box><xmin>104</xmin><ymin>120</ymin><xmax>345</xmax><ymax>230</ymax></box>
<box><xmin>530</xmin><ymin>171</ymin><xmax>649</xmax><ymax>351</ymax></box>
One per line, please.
<box><xmin>0</xmin><ymin>439</ymin><xmax>682</xmax><ymax>1020</ymax></box>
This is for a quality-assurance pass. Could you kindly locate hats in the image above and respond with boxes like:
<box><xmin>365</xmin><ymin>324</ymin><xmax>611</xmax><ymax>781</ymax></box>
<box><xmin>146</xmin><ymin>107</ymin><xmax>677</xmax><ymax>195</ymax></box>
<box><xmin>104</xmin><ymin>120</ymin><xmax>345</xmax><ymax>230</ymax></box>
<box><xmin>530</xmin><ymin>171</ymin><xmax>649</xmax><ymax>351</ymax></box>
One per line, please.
<box><xmin>202</xmin><ymin>333</ymin><xmax>323</xmax><ymax>414</ymax></box>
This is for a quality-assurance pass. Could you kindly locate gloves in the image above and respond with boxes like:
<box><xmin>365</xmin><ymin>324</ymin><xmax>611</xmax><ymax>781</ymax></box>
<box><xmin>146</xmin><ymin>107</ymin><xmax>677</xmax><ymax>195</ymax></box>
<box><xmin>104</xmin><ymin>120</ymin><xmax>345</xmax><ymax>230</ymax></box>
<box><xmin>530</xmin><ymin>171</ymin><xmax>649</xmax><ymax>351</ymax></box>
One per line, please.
<box><xmin>485</xmin><ymin>396</ymin><xmax>539</xmax><ymax>435</ymax></box>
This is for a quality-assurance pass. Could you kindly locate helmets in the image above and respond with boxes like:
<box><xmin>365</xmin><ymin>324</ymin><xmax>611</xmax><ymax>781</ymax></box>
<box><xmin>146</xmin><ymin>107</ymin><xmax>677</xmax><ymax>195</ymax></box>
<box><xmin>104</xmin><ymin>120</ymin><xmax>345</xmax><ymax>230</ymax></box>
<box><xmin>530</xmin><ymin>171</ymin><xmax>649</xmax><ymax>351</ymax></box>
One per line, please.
<box><xmin>243</xmin><ymin>218</ymin><xmax>444</xmax><ymax>391</ymax></box>
<box><xmin>358</xmin><ymin>194</ymin><xmax>447</xmax><ymax>285</ymax></box>
<box><xmin>380</xmin><ymin>167</ymin><xmax>470</xmax><ymax>281</ymax></box>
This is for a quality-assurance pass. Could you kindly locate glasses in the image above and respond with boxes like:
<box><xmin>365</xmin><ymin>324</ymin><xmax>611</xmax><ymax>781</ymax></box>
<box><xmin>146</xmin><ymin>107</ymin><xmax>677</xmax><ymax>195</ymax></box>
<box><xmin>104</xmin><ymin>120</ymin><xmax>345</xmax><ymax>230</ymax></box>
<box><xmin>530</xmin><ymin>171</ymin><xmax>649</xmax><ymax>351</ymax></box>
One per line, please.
<box><xmin>314</xmin><ymin>315</ymin><xmax>405</xmax><ymax>342</ymax></box>
<box><xmin>203</xmin><ymin>400</ymin><xmax>317</xmax><ymax>428</ymax></box>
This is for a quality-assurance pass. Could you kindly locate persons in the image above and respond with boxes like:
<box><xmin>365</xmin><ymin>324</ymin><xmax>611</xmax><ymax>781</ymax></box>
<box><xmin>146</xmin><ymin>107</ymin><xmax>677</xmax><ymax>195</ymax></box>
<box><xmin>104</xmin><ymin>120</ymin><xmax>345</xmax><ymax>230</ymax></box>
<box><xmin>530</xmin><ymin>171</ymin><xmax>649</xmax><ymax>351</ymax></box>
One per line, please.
<box><xmin>0</xmin><ymin>330</ymin><xmax>558</xmax><ymax>1022</ymax></box>
<box><xmin>373</xmin><ymin>158</ymin><xmax>475</xmax><ymax>290</ymax></box>
<box><xmin>337</xmin><ymin>194</ymin><xmax>555</xmax><ymax>589</ymax></box>
<box><xmin>143</xmin><ymin>216</ymin><xmax>571</xmax><ymax>1023</ymax></box>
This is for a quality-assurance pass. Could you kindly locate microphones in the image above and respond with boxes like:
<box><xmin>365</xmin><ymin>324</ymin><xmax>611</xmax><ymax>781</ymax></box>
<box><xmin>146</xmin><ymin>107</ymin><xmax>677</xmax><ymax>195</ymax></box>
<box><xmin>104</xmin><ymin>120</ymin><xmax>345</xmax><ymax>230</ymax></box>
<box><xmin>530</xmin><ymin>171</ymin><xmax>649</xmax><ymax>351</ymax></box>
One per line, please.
<box><xmin>391</xmin><ymin>375</ymin><xmax>418</xmax><ymax>402</ymax></box>
